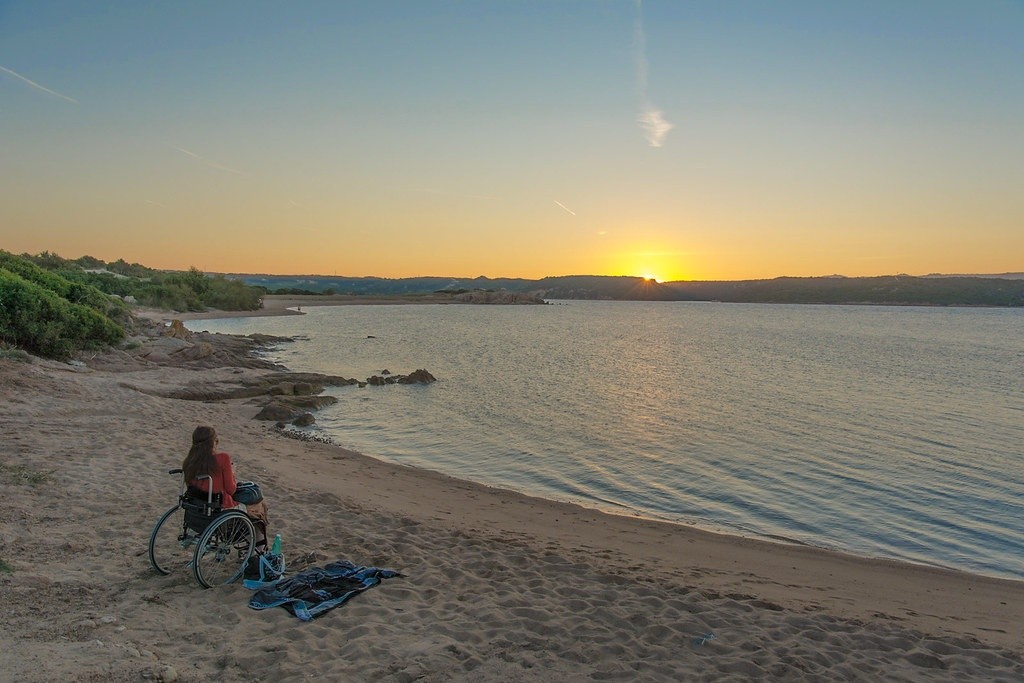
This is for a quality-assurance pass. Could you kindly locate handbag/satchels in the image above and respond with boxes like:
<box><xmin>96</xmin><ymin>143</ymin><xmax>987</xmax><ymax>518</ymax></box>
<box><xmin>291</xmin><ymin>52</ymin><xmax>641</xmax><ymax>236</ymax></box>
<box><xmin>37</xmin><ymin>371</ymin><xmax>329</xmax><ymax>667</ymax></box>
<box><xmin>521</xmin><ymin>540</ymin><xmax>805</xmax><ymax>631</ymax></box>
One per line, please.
<box><xmin>241</xmin><ymin>551</ymin><xmax>285</xmax><ymax>591</ymax></box>
<box><xmin>232</xmin><ymin>480</ymin><xmax>263</xmax><ymax>505</ymax></box>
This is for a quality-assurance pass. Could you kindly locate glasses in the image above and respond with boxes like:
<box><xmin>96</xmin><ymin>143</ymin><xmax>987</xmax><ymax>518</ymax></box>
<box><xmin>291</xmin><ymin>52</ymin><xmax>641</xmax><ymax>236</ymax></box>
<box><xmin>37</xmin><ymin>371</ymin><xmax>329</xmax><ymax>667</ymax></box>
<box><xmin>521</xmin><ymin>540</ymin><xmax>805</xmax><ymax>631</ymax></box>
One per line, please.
<box><xmin>215</xmin><ymin>438</ymin><xmax>219</xmax><ymax>445</ymax></box>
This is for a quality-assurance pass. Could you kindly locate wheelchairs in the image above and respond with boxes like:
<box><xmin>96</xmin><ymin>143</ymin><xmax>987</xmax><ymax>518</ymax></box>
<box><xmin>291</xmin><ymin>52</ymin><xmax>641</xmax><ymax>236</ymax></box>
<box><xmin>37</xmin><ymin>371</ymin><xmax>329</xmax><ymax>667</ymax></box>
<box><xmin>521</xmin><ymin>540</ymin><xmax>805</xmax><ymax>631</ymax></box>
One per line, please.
<box><xmin>148</xmin><ymin>468</ymin><xmax>283</xmax><ymax>591</ymax></box>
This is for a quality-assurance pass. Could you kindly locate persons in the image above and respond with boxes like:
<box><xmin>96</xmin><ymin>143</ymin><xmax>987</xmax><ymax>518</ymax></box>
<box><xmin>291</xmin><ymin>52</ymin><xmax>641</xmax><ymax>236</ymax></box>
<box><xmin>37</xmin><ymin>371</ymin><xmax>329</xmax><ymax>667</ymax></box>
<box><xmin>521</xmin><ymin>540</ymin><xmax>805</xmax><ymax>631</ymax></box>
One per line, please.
<box><xmin>181</xmin><ymin>424</ymin><xmax>268</xmax><ymax>561</ymax></box>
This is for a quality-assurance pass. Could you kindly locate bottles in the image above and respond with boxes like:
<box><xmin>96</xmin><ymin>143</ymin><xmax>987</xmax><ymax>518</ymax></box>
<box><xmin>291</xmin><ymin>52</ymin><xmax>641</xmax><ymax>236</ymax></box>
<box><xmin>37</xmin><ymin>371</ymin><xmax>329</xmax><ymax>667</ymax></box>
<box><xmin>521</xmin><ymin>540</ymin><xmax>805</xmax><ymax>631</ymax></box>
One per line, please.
<box><xmin>272</xmin><ymin>534</ymin><xmax>281</xmax><ymax>554</ymax></box>
